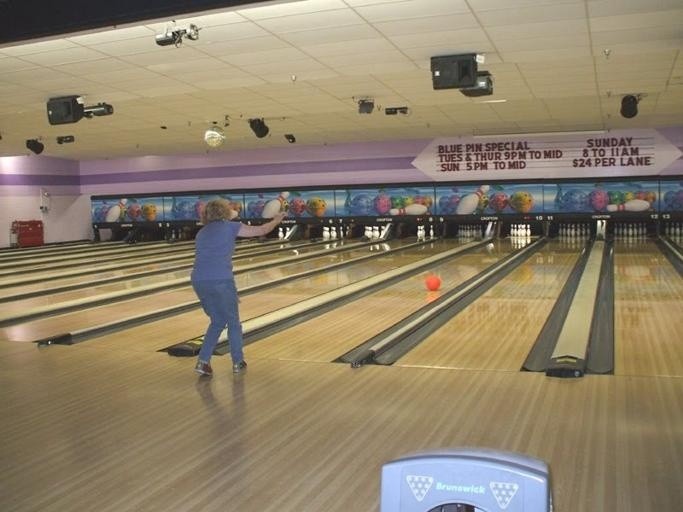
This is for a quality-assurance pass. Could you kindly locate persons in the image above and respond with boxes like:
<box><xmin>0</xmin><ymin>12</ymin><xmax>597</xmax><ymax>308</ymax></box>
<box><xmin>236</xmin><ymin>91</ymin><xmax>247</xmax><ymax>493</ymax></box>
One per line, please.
<box><xmin>193</xmin><ymin>374</ymin><xmax>248</xmax><ymax>471</ymax></box>
<box><xmin>187</xmin><ymin>198</ymin><xmax>287</xmax><ymax>375</ymax></box>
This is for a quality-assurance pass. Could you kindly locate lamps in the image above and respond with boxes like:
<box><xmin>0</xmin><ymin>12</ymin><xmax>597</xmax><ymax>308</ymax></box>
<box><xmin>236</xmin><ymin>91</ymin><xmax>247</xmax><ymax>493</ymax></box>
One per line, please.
<box><xmin>249</xmin><ymin>117</ymin><xmax>271</xmax><ymax>139</ymax></box>
<box><xmin>25</xmin><ymin>95</ymin><xmax>113</xmax><ymax>157</ymax></box>
<box><xmin>430</xmin><ymin>53</ymin><xmax>493</xmax><ymax>99</ymax></box>
<box><xmin>154</xmin><ymin>24</ymin><xmax>199</xmax><ymax>48</ymax></box>
<box><xmin>357</xmin><ymin>100</ymin><xmax>374</xmax><ymax>115</ymax></box>
<box><xmin>619</xmin><ymin>94</ymin><xmax>638</xmax><ymax>118</ymax></box>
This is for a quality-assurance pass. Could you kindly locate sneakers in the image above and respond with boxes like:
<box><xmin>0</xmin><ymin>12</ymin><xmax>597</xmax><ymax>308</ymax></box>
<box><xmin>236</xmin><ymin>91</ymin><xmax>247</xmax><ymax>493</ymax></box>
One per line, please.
<box><xmin>193</xmin><ymin>360</ymin><xmax>212</xmax><ymax>375</ymax></box>
<box><xmin>233</xmin><ymin>360</ymin><xmax>246</xmax><ymax>373</ymax></box>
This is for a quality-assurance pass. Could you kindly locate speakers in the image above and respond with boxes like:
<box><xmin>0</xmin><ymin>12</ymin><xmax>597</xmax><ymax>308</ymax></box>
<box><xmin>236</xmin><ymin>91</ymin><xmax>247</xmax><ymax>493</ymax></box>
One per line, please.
<box><xmin>430</xmin><ymin>52</ymin><xmax>478</xmax><ymax>90</ymax></box>
<box><xmin>359</xmin><ymin>101</ymin><xmax>373</xmax><ymax>113</ymax></box>
<box><xmin>459</xmin><ymin>71</ymin><xmax>494</xmax><ymax>97</ymax></box>
<box><xmin>46</xmin><ymin>93</ymin><xmax>83</xmax><ymax>125</ymax></box>
